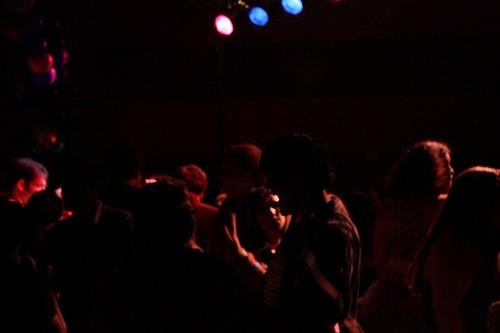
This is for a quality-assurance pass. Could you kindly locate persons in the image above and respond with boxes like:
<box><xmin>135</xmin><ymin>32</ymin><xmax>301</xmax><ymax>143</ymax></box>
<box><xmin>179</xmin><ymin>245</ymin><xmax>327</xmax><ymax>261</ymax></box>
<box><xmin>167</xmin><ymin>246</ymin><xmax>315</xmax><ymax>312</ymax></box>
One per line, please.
<box><xmin>4</xmin><ymin>44</ymin><xmax>500</xmax><ymax>333</ymax></box>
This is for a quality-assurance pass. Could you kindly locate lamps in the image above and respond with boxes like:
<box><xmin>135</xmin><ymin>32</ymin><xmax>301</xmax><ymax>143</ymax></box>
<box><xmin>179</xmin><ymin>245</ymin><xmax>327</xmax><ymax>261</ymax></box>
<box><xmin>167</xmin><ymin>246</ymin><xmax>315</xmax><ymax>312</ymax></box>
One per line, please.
<box><xmin>215</xmin><ymin>1</ymin><xmax>241</xmax><ymax>36</ymax></box>
<box><xmin>279</xmin><ymin>0</ymin><xmax>306</xmax><ymax>16</ymax></box>
<box><xmin>236</xmin><ymin>0</ymin><xmax>271</xmax><ymax>27</ymax></box>
